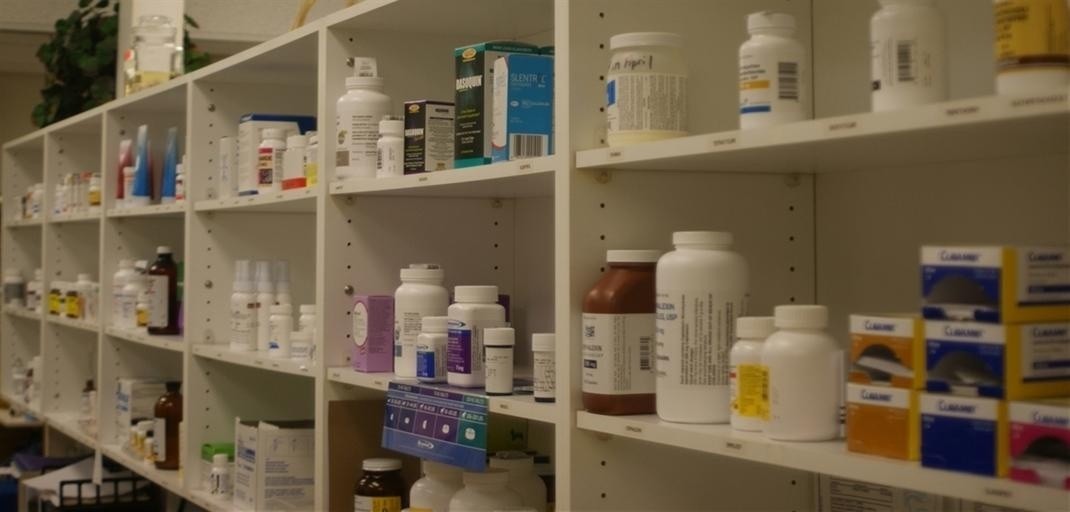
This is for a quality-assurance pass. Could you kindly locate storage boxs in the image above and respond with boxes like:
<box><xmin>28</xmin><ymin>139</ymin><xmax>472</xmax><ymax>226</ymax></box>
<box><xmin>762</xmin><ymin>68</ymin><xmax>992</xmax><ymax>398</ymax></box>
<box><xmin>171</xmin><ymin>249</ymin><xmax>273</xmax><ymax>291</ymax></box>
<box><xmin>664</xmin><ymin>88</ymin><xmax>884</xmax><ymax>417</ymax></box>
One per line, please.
<box><xmin>352</xmin><ymin>294</ymin><xmax>394</xmax><ymax>373</ymax></box>
<box><xmin>116</xmin><ymin>376</ymin><xmax>166</xmax><ymax>446</ymax></box>
<box><xmin>844</xmin><ymin>243</ymin><xmax>1068</xmax><ymax>491</ymax></box>
<box><xmin>404</xmin><ymin>39</ymin><xmax>554</xmax><ymax>175</ymax></box>
<box><xmin>233</xmin><ymin>414</ymin><xmax>315</xmax><ymax>511</ymax></box>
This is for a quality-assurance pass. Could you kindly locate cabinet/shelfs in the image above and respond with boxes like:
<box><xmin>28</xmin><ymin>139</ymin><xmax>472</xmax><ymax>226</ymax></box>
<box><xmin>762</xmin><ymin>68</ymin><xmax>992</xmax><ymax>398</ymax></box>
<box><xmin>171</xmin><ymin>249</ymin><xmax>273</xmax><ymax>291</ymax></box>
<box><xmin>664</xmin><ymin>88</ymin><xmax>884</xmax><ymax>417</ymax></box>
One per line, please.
<box><xmin>0</xmin><ymin>0</ymin><xmax>1070</xmax><ymax>511</ymax></box>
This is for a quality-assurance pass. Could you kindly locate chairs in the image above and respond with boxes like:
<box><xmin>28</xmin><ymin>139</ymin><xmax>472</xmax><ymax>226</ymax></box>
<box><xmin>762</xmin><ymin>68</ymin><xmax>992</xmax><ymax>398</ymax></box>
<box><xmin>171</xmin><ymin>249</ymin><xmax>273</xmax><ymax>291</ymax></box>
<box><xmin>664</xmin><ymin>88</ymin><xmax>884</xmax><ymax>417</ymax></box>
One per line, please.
<box><xmin>42</xmin><ymin>473</ymin><xmax>165</xmax><ymax>509</ymax></box>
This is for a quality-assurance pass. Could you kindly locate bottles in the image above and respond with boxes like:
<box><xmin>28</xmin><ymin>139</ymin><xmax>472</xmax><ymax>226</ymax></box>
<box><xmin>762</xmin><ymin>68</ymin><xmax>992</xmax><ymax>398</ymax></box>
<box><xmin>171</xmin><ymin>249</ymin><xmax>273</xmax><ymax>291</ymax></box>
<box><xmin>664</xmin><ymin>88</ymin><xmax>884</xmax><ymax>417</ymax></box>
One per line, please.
<box><xmin>129</xmin><ymin>379</ymin><xmax>183</xmax><ymax>469</ymax></box>
<box><xmin>603</xmin><ymin>31</ymin><xmax>688</xmax><ymax>148</ymax></box>
<box><xmin>334</xmin><ymin>77</ymin><xmax>405</xmax><ymax>182</ymax></box>
<box><xmin>765</xmin><ymin>302</ymin><xmax>842</xmax><ymax>443</ymax></box>
<box><xmin>230</xmin><ymin>260</ymin><xmax>315</xmax><ymax>362</ymax></box>
<box><xmin>113</xmin><ymin>245</ymin><xmax>180</xmax><ymax>336</ymax></box>
<box><xmin>12</xmin><ymin>356</ymin><xmax>41</xmax><ymax>409</ymax></box>
<box><xmin>4</xmin><ymin>267</ymin><xmax>41</xmax><ymax>313</ymax></box>
<box><xmin>210</xmin><ymin>452</ymin><xmax>230</xmax><ymax>498</ymax></box>
<box><xmin>122</xmin><ymin>166</ymin><xmax>138</xmax><ymax>205</ymax></box>
<box><xmin>728</xmin><ymin>314</ymin><xmax>776</xmax><ymax>431</ymax></box>
<box><xmin>54</xmin><ymin>171</ymin><xmax>101</xmax><ymax>214</ymax></box>
<box><xmin>25</xmin><ymin>182</ymin><xmax>43</xmax><ymax>218</ymax></box>
<box><xmin>869</xmin><ymin>1</ymin><xmax>951</xmax><ymax>112</ymax></box>
<box><xmin>653</xmin><ymin>227</ymin><xmax>751</xmax><ymax>426</ymax></box>
<box><xmin>48</xmin><ymin>273</ymin><xmax>98</xmax><ymax>322</ymax></box>
<box><xmin>991</xmin><ymin>0</ymin><xmax>1069</xmax><ymax>96</ymax></box>
<box><xmin>738</xmin><ymin>12</ymin><xmax>812</xmax><ymax>130</ymax></box>
<box><xmin>352</xmin><ymin>451</ymin><xmax>555</xmax><ymax>512</ymax></box>
<box><xmin>393</xmin><ymin>265</ymin><xmax>557</xmax><ymax>402</ymax></box>
<box><xmin>256</xmin><ymin>129</ymin><xmax>318</xmax><ymax>194</ymax></box>
<box><xmin>581</xmin><ymin>246</ymin><xmax>663</xmax><ymax>415</ymax></box>
<box><xmin>173</xmin><ymin>163</ymin><xmax>186</xmax><ymax>203</ymax></box>
<box><xmin>78</xmin><ymin>378</ymin><xmax>96</xmax><ymax>426</ymax></box>
